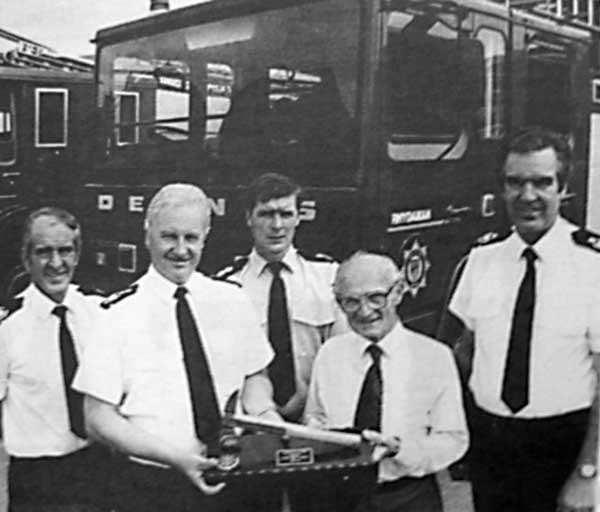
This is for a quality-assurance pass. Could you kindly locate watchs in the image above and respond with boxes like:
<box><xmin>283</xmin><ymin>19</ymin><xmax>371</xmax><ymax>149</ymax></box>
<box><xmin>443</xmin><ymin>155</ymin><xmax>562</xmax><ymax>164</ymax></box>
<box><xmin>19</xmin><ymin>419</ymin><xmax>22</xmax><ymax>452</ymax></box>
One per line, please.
<box><xmin>575</xmin><ymin>463</ymin><xmax>600</xmax><ymax>480</ymax></box>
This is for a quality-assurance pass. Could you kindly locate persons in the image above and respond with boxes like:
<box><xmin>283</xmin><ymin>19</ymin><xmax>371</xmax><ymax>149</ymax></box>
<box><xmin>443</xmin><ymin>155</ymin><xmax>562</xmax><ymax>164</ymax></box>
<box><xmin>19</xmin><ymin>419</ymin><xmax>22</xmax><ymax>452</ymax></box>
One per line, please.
<box><xmin>212</xmin><ymin>171</ymin><xmax>354</xmax><ymax>427</ymax></box>
<box><xmin>443</xmin><ymin>130</ymin><xmax>600</xmax><ymax>512</ymax></box>
<box><xmin>0</xmin><ymin>205</ymin><xmax>111</xmax><ymax>512</ymax></box>
<box><xmin>283</xmin><ymin>248</ymin><xmax>435</xmax><ymax>512</ymax></box>
<box><xmin>69</xmin><ymin>182</ymin><xmax>297</xmax><ymax>512</ymax></box>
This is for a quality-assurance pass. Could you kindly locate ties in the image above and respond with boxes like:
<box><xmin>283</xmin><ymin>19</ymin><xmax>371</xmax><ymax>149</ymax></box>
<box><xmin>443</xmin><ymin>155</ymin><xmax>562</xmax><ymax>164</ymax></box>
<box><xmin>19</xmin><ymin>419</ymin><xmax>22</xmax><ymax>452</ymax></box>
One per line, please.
<box><xmin>173</xmin><ymin>288</ymin><xmax>221</xmax><ymax>445</ymax></box>
<box><xmin>353</xmin><ymin>346</ymin><xmax>381</xmax><ymax>483</ymax></box>
<box><xmin>501</xmin><ymin>247</ymin><xmax>538</xmax><ymax>413</ymax></box>
<box><xmin>266</xmin><ymin>263</ymin><xmax>298</xmax><ymax>424</ymax></box>
<box><xmin>52</xmin><ymin>307</ymin><xmax>88</xmax><ymax>440</ymax></box>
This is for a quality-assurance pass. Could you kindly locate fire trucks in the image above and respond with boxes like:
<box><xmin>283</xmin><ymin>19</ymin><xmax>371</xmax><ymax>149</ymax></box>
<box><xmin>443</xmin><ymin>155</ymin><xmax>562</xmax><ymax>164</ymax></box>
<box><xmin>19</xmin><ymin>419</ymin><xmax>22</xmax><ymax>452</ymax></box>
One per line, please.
<box><xmin>0</xmin><ymin>28</ymin><xmax>161</xmax><ymax>324</ymax></box>
<box><xmin>85</xmin><ymin>1</ymin><xmax>599</xmax><ymax>344</ymax></box>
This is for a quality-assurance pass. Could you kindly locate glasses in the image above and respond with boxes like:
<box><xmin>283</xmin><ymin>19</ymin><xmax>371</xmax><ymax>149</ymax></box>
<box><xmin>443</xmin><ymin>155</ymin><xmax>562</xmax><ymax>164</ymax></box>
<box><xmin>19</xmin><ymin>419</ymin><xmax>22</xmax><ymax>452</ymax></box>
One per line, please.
<box><xmin>335</xmin><ymin>279</ymin><xmax>404</xmax><ymax>314</ymax></box>
